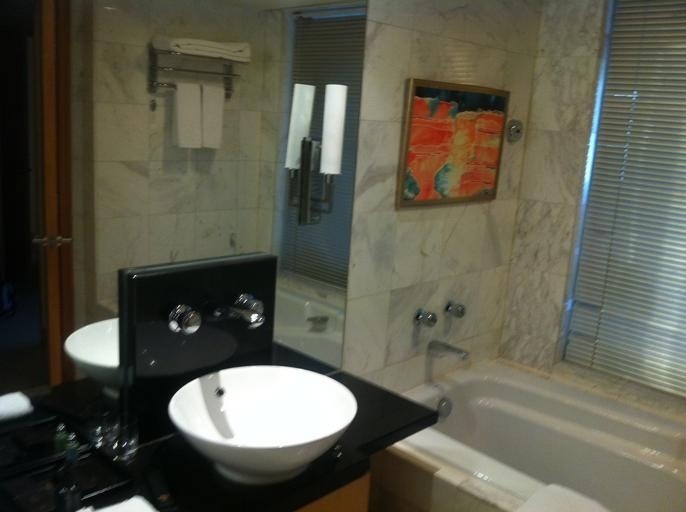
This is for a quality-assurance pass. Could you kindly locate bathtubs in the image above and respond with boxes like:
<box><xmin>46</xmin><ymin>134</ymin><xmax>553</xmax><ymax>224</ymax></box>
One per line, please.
<box><xmin>368</xmin><ymin>358</ymin><xmax>686</xmax><ymax>512</ymax></box>
<box><xmin>275</xmin><ymin>285</ymin><xmax>344</xmax><ymax>367</ymax></box>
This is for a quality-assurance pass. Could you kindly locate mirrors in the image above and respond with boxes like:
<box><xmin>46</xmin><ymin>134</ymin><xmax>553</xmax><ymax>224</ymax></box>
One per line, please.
<box><xmin>0</xmin><ymin>0</ymin><xmax>369</xmax><ymax>484</ymax></box>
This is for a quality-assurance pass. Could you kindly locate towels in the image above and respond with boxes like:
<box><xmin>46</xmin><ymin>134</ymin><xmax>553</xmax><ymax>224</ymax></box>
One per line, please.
<box><xmin>0</xmin><ymin>391</ymin><xmax>34</xmax><ymax>422</ymax></box>
<box><xmin>511</xmin><ymin>482</ymin><xmax>610</xmax><ymax>512</ymax></box>
<box><xmin>200</xmin><ymin>79</ymin><xmax>226</xmax><ymax>150</ymax></box>
<box><xmin>168</xmin><ymin>38</ymin><xmax>251</xmax><ymax>62</ymax></box>
<box><xmin>75</xmin><ymin>496</ymin><xmax>159</xmax><ymax>512</ymax></box>
<box><xmin>167</xmin><ymin>82</ymin><xmax>202</xmax><ymax>149</ymax></box>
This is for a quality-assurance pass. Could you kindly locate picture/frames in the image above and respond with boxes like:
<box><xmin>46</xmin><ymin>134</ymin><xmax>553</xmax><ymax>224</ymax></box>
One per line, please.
<box><xmin>395</xmin><ymin>77</ymin><xmax>511</xmax><ymax>210</ymax></box>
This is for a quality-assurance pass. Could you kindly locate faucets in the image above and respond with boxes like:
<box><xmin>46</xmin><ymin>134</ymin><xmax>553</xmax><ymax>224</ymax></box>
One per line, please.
<box><xmin>427</xmin><ymin>316</ymin><xmax>470</xmax><ymax>364</ymax></box>
<box><xmin>229</xmin><ymin>292</ymin><xmax>264</xmax><ymax>330</ymax></box>
<box><xmin>167</xmin><ymin>303</ymin><xmax>203</xmax><ymax>335</ymax></box>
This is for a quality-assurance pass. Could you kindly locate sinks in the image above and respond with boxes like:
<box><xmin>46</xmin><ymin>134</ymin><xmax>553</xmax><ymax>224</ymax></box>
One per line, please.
<box><xmin>167</xmin><ymin>363</ymin><xmax>360</xmax><ymax>487</ymax></box>
<box><xmin>61</xmin><ymin>317</ymin><xmax>122</xmax><ymax>400</ymax></box>
